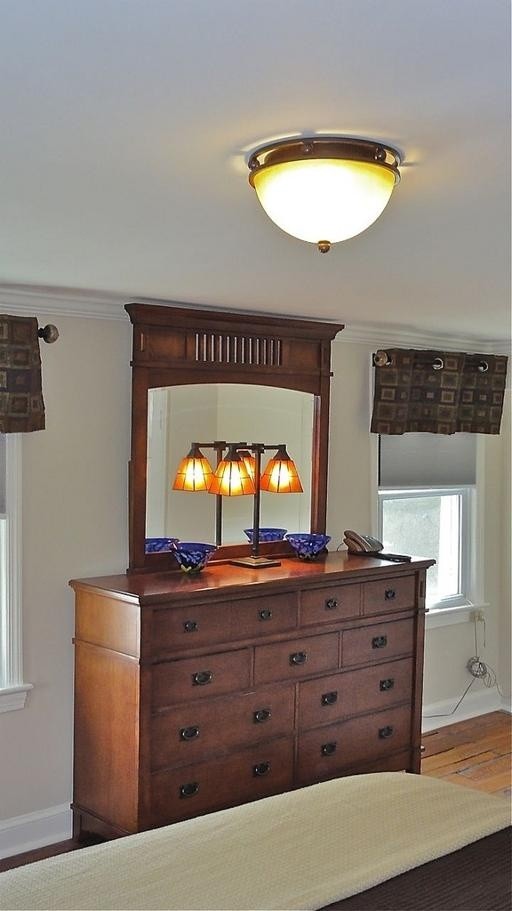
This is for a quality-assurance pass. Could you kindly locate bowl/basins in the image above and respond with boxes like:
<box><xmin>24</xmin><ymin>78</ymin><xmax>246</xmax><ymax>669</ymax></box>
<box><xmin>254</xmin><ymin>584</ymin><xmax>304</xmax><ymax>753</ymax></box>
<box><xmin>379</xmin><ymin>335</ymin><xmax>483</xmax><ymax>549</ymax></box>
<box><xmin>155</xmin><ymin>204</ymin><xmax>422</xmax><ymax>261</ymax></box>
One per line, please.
<box><xmin>167</xmin><ymin>542</ymin><xmax>220</xmax><ymax>575</ymax></box>
<box><xmin>145</xmin><ymin>537</ymin><xmax>179</xmax><ymax>553</ymax></box>
<box><xmin>243</xmin><ymin>528</ymin><xmax>288</xmax><ymax>543</ymax></box>
<box><xmin>283</xmin><ymin>532</ymin><xmax>332</xmax><ymax>563</ymax></box>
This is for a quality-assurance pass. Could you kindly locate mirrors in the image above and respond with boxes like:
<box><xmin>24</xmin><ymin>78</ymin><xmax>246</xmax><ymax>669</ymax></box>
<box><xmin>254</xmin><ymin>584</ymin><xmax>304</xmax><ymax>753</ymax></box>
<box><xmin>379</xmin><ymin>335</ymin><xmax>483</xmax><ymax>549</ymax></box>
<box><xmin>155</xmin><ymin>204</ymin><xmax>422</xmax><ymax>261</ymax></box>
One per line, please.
<box><xmin>124</xmin><ymin>302</ymin><xmax>345</xmax><ymax>574</ymax></box>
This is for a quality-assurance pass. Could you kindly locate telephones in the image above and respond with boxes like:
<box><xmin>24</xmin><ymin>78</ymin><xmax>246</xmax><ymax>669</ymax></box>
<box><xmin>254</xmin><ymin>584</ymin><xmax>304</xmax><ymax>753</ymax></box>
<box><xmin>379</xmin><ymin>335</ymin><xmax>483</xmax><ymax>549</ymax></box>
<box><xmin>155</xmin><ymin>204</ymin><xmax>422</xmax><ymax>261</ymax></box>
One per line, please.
<box><xmin>342</xmin><ymin>530</ymin><xmax>384</xmax><ymax>552</ymax></box>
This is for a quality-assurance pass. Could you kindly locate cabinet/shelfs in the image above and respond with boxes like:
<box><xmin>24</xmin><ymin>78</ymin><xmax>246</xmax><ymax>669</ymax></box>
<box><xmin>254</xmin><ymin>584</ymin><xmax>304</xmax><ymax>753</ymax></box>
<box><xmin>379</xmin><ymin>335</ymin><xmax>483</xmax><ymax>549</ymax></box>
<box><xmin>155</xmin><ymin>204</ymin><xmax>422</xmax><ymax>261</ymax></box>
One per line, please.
<box><xmin>68</xmin><ymin>550</ymin><xmax>436</xmax><ymax>848</ymax></box>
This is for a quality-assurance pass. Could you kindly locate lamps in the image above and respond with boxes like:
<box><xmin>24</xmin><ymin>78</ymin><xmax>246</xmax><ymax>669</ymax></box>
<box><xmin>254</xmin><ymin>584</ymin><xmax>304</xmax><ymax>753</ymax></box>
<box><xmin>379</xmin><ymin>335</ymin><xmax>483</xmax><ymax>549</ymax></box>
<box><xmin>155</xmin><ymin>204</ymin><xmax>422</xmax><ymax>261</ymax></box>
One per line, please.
<box><xmin>207</xmin><ymin>441</ymin><xmax>304</xmax><ymax>570</ymax></box>
<box><xmin>247</xmin><ymin>136</ymin><xmax>403</xmax><ymax>254</ymax></box>
<box><xmin>172</xmin><ymin>439</ymin><xmax>256</xmax><ymax>548</ymax></box>
